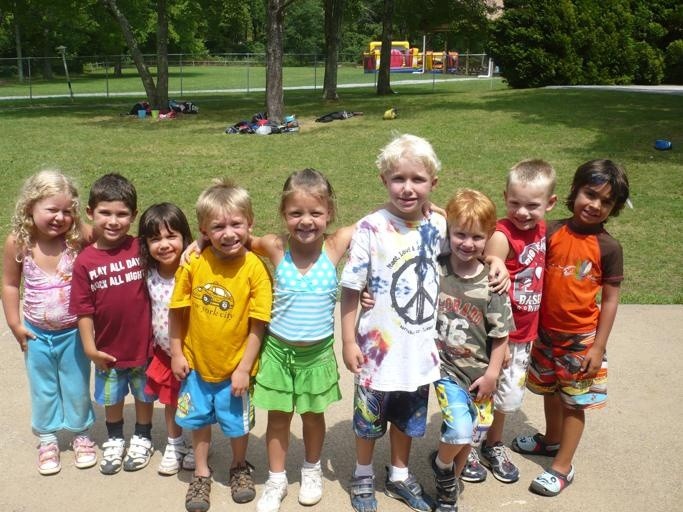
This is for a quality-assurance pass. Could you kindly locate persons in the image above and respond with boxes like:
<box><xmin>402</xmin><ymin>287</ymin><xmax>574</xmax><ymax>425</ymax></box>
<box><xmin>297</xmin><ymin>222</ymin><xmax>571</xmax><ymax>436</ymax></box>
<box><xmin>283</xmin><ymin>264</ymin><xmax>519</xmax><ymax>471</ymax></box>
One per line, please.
<box><xmin>360</xmin><ymin>188</ymin><xmax>517</xmax><ymax>512</ymax></box>
<box><xmin>512</xmin><ymin>157</ymin><xmax>630</xmax><ymax>497</ymax></box>
<box><xmin>165</xmin><ymin>175</ymin><xmax>272</xmax><ymax>512</ymax></box>
<box><xmin>458</xmin><ymin>158</ymin><xmax>558</xmax><ymax>484</ymax></box>
<box><xmin>67</xmin><ymin>172</ymin><xmax>158</xmax><ymax>476</ymax></box>
<box><xmin>177</xmin><ymin>167</ymin><xmax>447</xmax><ymax>512</ymax></box>
<box><xmin>338</xmin><ymin>132</ymin><xmax>513</xmax><ymax>512</ymax></box>
<box><xmin>136</xmin><ymin>202</ymin><xmax>212</xmax><ymax>476</ymax></box>
<box><xmin>0</xmin><ymin>169</ymin><xmax>98</xmax><ymax>475</ymax></box>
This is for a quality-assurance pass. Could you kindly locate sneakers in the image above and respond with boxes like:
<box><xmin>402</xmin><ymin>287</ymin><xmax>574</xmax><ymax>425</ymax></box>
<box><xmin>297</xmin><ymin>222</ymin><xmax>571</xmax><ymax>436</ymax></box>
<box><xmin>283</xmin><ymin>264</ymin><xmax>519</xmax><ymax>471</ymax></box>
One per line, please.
<box><xmin>383</xmin><ymin>464</ymin><xmax>435</xmax><ymax>512</ymax></box>
<box><xmin>298</xmin><ymin>465</ymin><xmax>324</xmax><ymax>506</ymax></box>
<box><xmin>70</xmin><ymin>436</ymin><xmax>97</xmax><ymax>469</ymax></box>
<box><xmin>184</xmin><ymin>465</ymin><xmax>213</xmax><ymax>512</ymax></box>
<box><xmin>157</xmin><ymin>439</ymin><xmax>186</xmax><ymax>476</ymax></box>
<box><xmin>229</xmin><ymin>460</ymin><xmax>257</xmax><ymax>503</ymax></box>
<box><xmin>256</xmin><ymin>475</ymin><xmax>288</xmax><ymax>512</ymax></box>
<box><xmin>459</xmin><ymin>440</ymin><xmax>486</xmax><ymax>482</ymax></box>
<box><xmin>478</xmin><ymin>438</ymin><xmax>520</xmax><ymax>483</ymax></box>
<box><xmin>99</xmin><ymin>438</ymin><xmax>125</xmax><ymax>474</ymax></box>
<box><xmin>428</xmin><ymin>449</ymin><xmax>459</xmax><ymax>501</ymax></box>
<box><xmin>433</xmin><ymin>478</ymin><xmax>460</xmax><ymax>511</ymax></box>
<box><xmin>123</xmin><ymin>435</ymin><xmax>153</xmax><ymax>471</ymax></box>
<box><xmin>36</xmin><ymin>440</ymin><xmax>62</xmax><ymax>476</ymax></box>
<box><xmin>182</xmin><ymin>439</ymin><xmax>215</xmax><ymax>469</ymax></box>
<box><xmin>348</xmin><ymin>465</ymin><xmax>377</xmax><ymax>512</ymax></box>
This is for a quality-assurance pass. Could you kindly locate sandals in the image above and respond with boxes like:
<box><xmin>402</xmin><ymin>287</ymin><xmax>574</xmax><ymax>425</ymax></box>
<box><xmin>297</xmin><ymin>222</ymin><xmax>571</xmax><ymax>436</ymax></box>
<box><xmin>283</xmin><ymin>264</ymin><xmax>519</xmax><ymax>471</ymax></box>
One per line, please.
<box><xmin>530</xmin><ymin>461</ymin><xmax>576</xmax><ymax>497</ymax></box>
<box><xmin>508</xmin><ymin>432</ymin><xmax>561</xmax><ymax>456</ymax></box>
